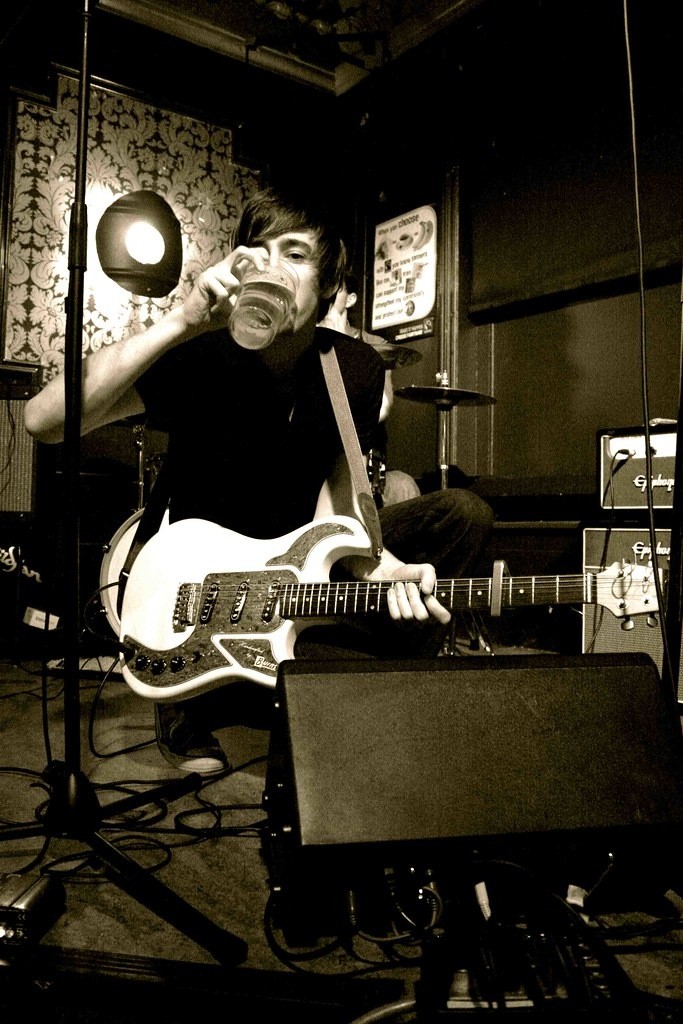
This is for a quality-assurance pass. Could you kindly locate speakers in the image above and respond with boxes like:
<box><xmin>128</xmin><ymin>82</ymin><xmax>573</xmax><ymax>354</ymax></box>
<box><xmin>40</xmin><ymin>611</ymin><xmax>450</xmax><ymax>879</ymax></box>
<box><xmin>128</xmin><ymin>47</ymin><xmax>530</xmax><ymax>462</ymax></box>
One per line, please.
<box><xmin>262</xmin><ymin>650</ymin><xmax>683</xmax><ymax>948</ymax></box>
<box><xmin>0</xmin><ymin>381</ymin><xmax>42</xmax><ymax>517</ymax></box>
<box><xmin>580</xmin><ymin>528</ymin><xmax>683</xmax><ymax>705</ymax></box>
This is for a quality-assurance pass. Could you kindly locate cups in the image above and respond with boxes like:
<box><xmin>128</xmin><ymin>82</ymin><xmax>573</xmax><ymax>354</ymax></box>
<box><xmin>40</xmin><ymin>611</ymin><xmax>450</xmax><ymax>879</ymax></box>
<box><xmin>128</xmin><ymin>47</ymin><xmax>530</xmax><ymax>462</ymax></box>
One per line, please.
<box><xmin>228</xmin><ymin>256</ymin><xmax>300</xmax><ymax>350</ymax></box>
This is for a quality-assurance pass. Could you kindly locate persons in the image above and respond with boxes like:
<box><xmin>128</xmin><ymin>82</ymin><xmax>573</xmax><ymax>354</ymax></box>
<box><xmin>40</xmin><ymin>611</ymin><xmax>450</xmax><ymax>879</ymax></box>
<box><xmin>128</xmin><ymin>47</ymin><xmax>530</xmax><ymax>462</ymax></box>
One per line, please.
<box><xmin>21</xmin><ymin>182</ymin><xmax>494</xmax><ymax>773</ymax></box>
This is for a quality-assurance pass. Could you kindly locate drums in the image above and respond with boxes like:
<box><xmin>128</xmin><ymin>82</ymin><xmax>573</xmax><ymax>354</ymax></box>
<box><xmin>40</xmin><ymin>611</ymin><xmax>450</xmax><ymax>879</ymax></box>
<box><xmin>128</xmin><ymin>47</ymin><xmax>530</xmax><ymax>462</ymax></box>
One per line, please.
<box><xmin>98</xmin><ymin>505</ymin><xmax>145</xmax><ymax>640</ymax></box>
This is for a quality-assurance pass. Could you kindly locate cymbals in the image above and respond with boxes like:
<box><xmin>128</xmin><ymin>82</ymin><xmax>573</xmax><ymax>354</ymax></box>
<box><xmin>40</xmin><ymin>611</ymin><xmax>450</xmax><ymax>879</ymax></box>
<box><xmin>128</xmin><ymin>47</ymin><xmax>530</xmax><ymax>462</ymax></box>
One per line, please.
<box><xmin>393</xmin><ymin>383</ymin><xmax>497</xmax><ymax>409</ymax></box>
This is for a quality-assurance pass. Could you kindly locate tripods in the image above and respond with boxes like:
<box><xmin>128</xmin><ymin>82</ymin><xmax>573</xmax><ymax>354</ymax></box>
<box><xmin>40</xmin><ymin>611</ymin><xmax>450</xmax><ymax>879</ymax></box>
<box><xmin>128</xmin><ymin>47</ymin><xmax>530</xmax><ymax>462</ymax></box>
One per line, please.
<box><xmin>0</xmin><ymin>0</ymin><xmax>250</xmax><ymax>969</ymax></box>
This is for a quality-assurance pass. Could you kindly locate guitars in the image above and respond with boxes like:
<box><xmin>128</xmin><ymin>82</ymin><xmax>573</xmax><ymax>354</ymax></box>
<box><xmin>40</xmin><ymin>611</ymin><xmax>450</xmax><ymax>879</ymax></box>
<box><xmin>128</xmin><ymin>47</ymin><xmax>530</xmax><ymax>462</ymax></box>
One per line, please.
<box><xmin>116</xmin><ymin>514</ymin><xmax>665</xmax><ymax>704</ymax></box>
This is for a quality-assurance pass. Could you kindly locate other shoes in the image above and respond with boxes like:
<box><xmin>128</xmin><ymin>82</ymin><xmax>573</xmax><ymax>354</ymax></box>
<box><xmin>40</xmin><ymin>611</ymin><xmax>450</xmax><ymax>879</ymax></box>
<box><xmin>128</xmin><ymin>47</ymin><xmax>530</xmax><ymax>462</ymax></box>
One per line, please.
<box><xmin>154</xmin><ymin>701</ymin><xmax>227</xmax><ymax>772</ymax></box>
